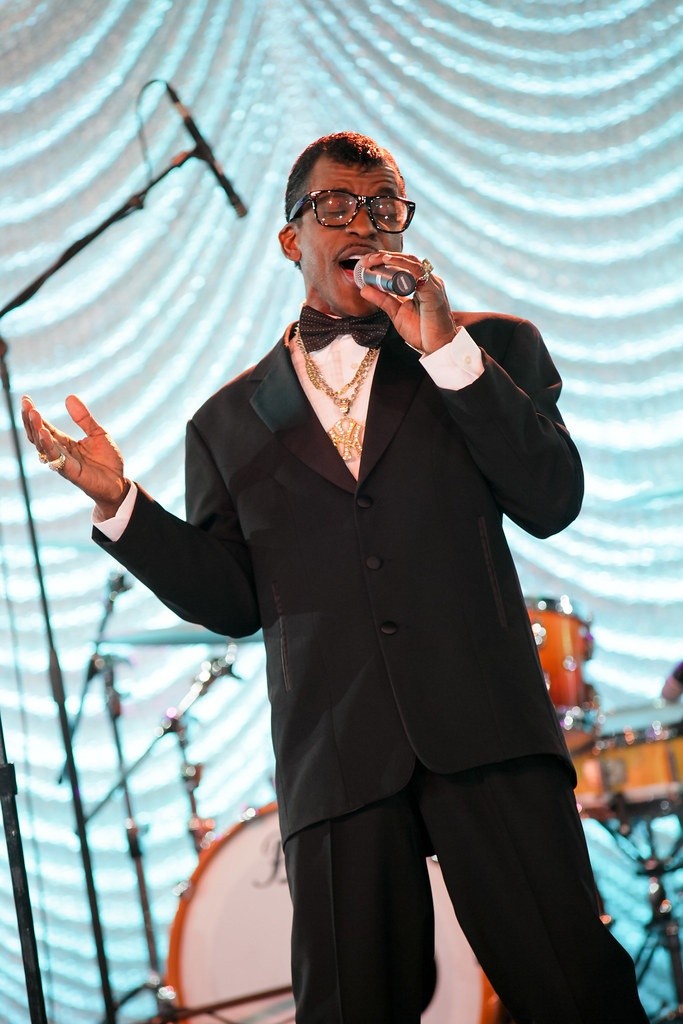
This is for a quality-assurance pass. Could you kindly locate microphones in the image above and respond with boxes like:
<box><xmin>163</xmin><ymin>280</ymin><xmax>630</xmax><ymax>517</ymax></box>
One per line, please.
<box><xmin>164</xmin><ymin>80</ymin><xmax>249</xmax><ymax>215</ymax></box>
<box><xmin>353</xmin><ymin>257</ymin><xmax>417</xmax><ymax>296</ymax></box>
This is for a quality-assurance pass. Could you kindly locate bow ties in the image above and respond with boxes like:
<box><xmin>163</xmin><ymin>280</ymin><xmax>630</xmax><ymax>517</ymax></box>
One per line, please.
<box><xmin>300</xmin><ymin>306</ymin><xmax>389</xmax><ymax>351</ymax></box>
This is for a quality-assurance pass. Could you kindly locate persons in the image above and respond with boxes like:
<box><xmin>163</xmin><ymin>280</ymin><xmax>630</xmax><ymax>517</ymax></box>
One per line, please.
<box><xmin>661</xmin><ymin>661</ymin><xmax>683</xmax><ymax>702</ymax></box>
<box><xmin>21</xmin><ymin>131</ymin><xmax>649</xmax><ymax>1024</ymax></box>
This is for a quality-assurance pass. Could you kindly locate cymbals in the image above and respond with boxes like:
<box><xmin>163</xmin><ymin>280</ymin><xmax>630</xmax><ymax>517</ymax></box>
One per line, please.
<box><xmin>84</xmin><ymin>621</ymin><xmax>266</xmax><ymax>644</ymax></box>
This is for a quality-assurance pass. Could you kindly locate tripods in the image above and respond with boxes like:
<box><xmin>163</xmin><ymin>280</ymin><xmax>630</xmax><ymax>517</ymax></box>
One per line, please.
<box><xmin>92</xmin><ymin>588</ymin><xmax>228</xmax><ymax>1024</ymax></box>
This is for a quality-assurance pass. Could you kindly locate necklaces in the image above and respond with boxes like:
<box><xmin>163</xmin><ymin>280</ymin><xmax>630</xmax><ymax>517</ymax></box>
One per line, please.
<box><xmin>296</xmin><ymin>325</ymin><xmax>377</xmax><ymax>459</ymax></box>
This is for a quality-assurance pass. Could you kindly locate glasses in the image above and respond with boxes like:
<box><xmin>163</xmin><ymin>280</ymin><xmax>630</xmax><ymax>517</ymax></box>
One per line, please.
<box><xmin>290</xmin><ymin>187</ymin><xmax>416</xmax><ymax>233</ymax></box>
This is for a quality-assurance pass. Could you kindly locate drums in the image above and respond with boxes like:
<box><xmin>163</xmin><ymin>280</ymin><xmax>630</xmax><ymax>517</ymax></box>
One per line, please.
<box><xmin>569</xmin><ymin>719</ymin><xmax>683</xmax><ymax>817</ymax></box>
<box><xmin>519</xmin><ymin>595</ymin><xmax>599</xmax><ymax>756</ymax></box>
<box><xmin>161</xmin><ymin>796</ymin><xmax>509</xmax><ymax>1024</ymax></box>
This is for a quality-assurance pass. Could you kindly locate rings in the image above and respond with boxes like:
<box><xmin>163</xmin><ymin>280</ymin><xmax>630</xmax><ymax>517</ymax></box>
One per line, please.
<box><xmin>417</xmin><ymin>269</ymin><xmax>430</xmax><ymax>285</ymax></box>
<box><xmin>38</xmin><ymin>453</ymin><xmax>48</xmax><ymax>463</ymax></box>
<box><xmin>422</xmin><ymin>259</ymin><xmax>433</xmax><ymax>272</ymax></box>
<box><xmin>49</xmin><ymin>453</ymin><xmax>65</xmax><ymax>469</ymax></box>
